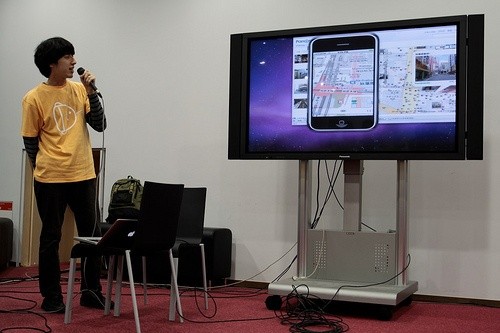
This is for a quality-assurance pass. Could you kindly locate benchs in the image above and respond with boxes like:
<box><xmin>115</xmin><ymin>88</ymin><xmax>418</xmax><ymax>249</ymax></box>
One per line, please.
<box><xmin>101</xmin><ymin>221</ymin><xmax>232</xmax><ymax>286</ymax></box>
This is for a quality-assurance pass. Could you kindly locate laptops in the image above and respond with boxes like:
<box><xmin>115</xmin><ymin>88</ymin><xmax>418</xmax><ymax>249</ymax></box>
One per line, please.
<box><xmin>72</xmin><ymin>219</ymin><xmax>138</xmax><ymax>246</ymax></box>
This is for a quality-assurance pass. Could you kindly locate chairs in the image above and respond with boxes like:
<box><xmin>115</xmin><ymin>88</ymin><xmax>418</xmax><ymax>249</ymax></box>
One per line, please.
<box><xmin>64</xmin><ymin>181</ymin><xmax>208</xmax><ymax>333</ymax></box>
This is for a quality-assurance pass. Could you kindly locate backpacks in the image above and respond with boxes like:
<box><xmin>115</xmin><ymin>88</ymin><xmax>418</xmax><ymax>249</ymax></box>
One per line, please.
<box><xmin>106</xmin><ymin>176</ymin><xmax>143</xmax><ymax>224</ymax></box>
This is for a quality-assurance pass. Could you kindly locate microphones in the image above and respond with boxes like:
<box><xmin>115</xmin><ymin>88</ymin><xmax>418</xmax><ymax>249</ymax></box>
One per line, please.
<box><xmin>78</xmin><ymin>67</ymin><xmax>103</xmax><ymax>98</ymax></box>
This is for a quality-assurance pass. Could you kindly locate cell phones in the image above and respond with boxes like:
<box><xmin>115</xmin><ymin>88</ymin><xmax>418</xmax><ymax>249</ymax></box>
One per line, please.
<box><xmin>309</xmin><ymin>34</ymin><xmax>379</xmax><ymax>132</ymax></box>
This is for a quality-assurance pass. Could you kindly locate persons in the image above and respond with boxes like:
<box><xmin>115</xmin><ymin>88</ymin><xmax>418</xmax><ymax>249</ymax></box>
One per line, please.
<box><xmin>20</xmin><ymin>37</ymin><xmax>115</xmax><ymax>314</ymax></box>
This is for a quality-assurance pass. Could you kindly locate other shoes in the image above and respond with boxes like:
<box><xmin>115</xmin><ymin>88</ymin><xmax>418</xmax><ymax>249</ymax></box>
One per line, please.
<box><xmin>42</xmin><ymin>297</ymin><xmax>66</xmax><ymax>314</ymax></box>
<box><xmin>80</xmin><ymin>294</ymin><xmax>115</xmax><ymax>309</ymax></box>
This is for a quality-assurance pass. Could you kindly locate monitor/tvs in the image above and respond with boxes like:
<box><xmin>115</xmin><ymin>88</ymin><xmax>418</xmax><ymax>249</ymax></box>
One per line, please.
<box><xmin>228</xmin><ymin>14</ymin><xmax>486</xmax><ymax>161</ymax></box>
